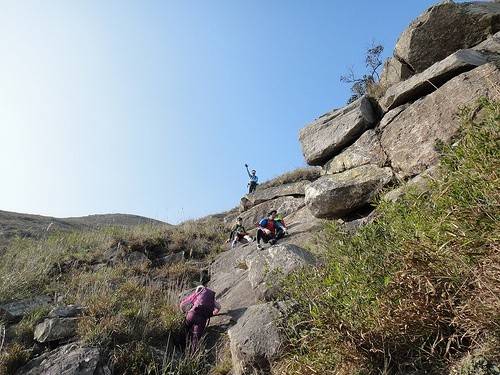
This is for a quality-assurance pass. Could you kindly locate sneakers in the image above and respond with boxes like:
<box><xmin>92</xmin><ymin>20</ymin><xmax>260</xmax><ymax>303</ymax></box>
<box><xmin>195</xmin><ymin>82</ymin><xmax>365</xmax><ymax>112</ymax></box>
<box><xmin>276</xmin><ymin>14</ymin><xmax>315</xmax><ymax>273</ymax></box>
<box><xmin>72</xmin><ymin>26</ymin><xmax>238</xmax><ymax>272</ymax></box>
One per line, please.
<box><xmin>268</xmin><ymin>239</ymin><xmax>276</xmax><ymax>245</ymax></box>
<box><xmin>257</xmin><ymin>244</ymin><xmax>263</xmax><ymax>250</ymax></box>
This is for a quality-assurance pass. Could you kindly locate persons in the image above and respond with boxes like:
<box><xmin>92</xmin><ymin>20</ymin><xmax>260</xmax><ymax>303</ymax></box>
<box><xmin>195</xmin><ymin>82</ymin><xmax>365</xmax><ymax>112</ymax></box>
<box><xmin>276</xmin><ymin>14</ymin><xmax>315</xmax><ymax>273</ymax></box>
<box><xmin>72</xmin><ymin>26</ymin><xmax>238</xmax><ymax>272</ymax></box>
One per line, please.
<box><xmin>245</xmin><ymin>164</ymin><xmax>258</xmax><ymax>194</ymax></box>
<box><xmin>256</xmin><ymin>209</ymin><xmax>289</xmax><ymax>251</ymax></box>
<box><xmin>226</xmin><ymin>217</ymin><xmax>257</xmax><ymax>248</ymax></box>
<box><xmin>179</xmin><ymin>284</ymin><xmax>222</xmax><ymax>352</ymax></box>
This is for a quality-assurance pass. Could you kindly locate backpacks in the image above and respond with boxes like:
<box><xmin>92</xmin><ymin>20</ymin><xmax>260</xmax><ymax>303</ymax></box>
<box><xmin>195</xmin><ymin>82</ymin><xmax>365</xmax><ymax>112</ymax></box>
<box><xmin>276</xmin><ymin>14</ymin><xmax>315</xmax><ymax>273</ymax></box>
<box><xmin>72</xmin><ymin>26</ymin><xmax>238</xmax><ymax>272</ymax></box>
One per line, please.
<box><xmin>189</xmin><ymin>285</ymin><xmax>216</xmax><ymax>317</ymax></box>
<box><xmin>235</xmin><ymin>224</ymin><xmax>245</xmax><ymax>238</ymax></box>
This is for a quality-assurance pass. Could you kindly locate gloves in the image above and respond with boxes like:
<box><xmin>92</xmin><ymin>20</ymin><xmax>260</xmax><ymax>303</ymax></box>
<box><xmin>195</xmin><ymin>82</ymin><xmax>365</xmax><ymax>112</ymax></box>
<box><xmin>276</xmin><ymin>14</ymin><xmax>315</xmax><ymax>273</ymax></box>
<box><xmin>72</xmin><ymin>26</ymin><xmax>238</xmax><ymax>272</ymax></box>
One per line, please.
<box><xmin>264</xmin><ymin>228</ymin><xmax>271</xmax><ymax>234</ymax></box>
<box><xmin>282</xmin><ymin>227</ymin><xmax>288</xmax><ymax>233</ymax></box>
